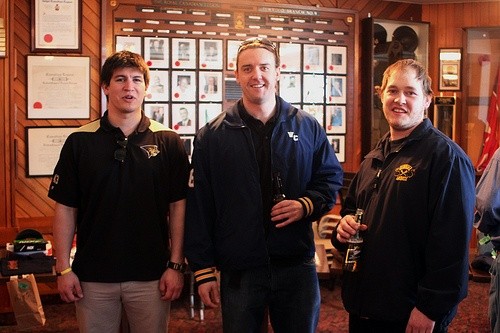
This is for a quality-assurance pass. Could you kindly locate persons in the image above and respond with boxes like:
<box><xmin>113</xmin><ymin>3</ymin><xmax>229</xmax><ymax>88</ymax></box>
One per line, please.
<box><xmin>178</xmin><ymin>107</ymin><xmax>191</xmax><ymax>126</ymax></box>
<box><xmin>204</xmin><ymin>78</ymin><xmax>217</xmax><ymax>94</ymax></box>
<box><xmin>185</xmin><ymin>38</ymin><xmax>343</xmax><ymax>333</ymax></box>
<box><xmin>332</xmin><ymin>81</ymin><xmax>342</xmax><ymax>97</ymax></box>
<box><xmin>150</xmin><ymin>40</ymin><xmax>164</xmax><ymax>60</ymax></box>
<box><xmin>473</xmin><ymin>147</ymin><xmax>500</xmax><ymax>333</ymax></box>
<box><xmin>177</xmin><ymin>78</ymin><xmax>191</xmax><ymax>94</ymax></box>
<box><xmin>331</xmin><ymin>59</ymin><xmax>475</xmax><ymax>333</ymax></box>
<box><xmin>48</xmin><ymin>50</ymin><xmax>190</xmax><ymax>333</ymax></box>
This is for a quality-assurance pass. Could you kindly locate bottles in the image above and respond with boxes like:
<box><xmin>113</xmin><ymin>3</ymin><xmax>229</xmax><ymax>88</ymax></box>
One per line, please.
<box><xmin>342</xmin><ymin>208</ymin><xmax>365</xmax><ymax>272</ymax></box>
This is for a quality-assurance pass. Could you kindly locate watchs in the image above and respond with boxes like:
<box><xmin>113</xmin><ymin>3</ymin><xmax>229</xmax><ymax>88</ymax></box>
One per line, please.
<box><xmin>166</xmin><ymin>260</ymin><xmax>187</xmax><ymax>273</ymax></box>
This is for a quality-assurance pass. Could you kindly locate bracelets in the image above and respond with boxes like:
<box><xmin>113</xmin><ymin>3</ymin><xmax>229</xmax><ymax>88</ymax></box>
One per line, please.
<box><xmin>56</xmin><ymin>266</ymin><xmax>72</xmax><ymax>276</ymax></box>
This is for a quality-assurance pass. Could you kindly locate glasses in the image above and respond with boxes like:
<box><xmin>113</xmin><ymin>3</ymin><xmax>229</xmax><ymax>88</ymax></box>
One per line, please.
<box><xmin>236</xmin><ymin>37</ymin><xmax>278</xmax><ymax>70</ymax></box>
<box><xmin>114</xmin><ymin>136</ymin><xmax>128</xmax><ymax>160</ymax></box>
